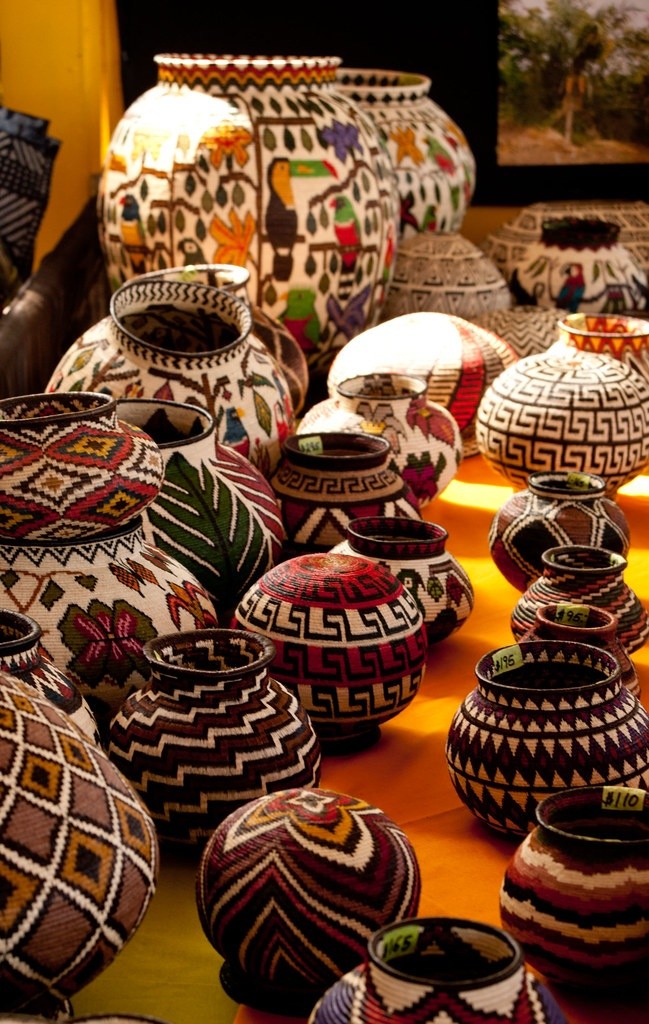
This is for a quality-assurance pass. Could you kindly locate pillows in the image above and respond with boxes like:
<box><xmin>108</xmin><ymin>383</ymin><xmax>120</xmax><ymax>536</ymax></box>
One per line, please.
<box><xmin>0</xmin><ymin>106</ymin><xmax>63</xmax><ymax>280</ymax></box>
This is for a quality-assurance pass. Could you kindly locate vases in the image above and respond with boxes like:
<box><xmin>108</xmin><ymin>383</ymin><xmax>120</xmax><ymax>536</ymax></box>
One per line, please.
<box><xmin>0</xmin><ymin>49</ymin><xmax>649</xmax><ymax>1023</ymax></box>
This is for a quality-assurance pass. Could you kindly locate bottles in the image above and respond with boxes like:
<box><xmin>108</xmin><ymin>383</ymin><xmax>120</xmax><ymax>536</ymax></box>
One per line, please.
<box><xmin>0</xmin><ymin>54</ymin><xmax>649</xmax><ymax>1024</ymax></box>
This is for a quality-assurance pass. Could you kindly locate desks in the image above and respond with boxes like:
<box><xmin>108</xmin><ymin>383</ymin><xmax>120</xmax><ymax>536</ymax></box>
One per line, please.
<box><xmin>74</xmin><ymin>204</ymin><xmax>649</xmax><ymax>1024</ymax></box>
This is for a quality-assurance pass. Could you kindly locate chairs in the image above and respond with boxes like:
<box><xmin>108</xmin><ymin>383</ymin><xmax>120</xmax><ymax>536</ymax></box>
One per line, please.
<box><xmin>0</xmin><ymin>195</ymin><xmax>110</xmax><ymax>401</ymax></box>
